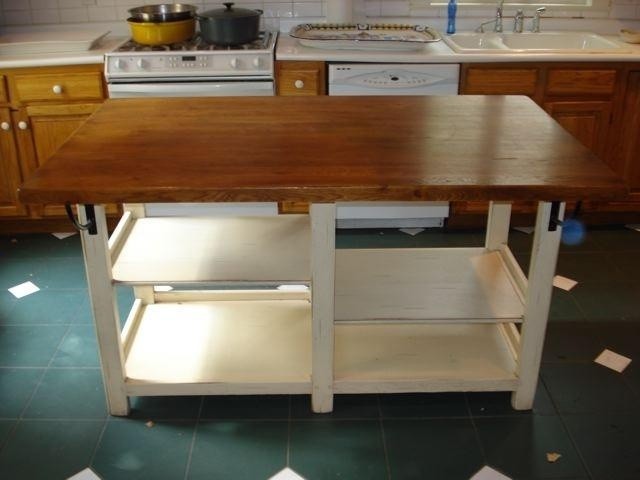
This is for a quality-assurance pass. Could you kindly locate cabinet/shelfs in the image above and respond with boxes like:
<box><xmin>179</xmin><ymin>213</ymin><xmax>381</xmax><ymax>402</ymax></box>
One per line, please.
<box><xmin>0</xmin><ymin>65</ymin><xmax>124</xmax><ymax>232</ymax></box>
<box><xmin>450</xmin><ymin>62</ymin><xmax>620</xmax><ymax>223</ymax></box>
<box><xmin>276</xmin><ymin>61</ymin><xmax>327</xmax><ymax>213</ymax></box>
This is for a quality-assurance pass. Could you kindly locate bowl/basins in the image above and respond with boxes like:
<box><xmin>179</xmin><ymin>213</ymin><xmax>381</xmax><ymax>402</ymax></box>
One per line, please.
<box><xmin>127</xmin><ymin>2</ymin><xmax>198</xmax><ymax>44</ymax></box>
<box><xmin>619</xmin><ymin>27</ymin><xmax>640</xmax><ymax>44</ymax></box>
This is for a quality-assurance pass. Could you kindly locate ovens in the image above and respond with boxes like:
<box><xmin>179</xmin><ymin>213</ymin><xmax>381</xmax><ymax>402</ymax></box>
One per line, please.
<box><xmin>109</xmin><ymin>77</ymin><xmax>275</xmax><ymax>217</ymax></box>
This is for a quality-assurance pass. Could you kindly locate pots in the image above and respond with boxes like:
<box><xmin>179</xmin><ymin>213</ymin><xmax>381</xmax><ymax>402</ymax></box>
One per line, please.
<box><xmin>194</xmin><ymin>3</ymin><xmax>263</xmax><ymax>42</ymax></box>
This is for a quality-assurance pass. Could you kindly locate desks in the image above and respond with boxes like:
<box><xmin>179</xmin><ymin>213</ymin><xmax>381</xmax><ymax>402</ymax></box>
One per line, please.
<box><xmin>15</xmin><ymin>96</ymin><xmax>629</xmax><ymax>416</ymax></box>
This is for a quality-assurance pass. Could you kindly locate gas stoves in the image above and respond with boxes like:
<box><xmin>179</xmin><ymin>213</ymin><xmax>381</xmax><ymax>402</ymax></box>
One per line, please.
<box><xmin>105</xmin><ymin>28</ymin><xmax>277</xmax><ymax>80</ymax></box>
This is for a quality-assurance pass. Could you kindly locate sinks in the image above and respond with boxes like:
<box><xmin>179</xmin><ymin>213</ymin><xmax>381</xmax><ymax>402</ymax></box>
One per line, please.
<box><xmin>500</xmin><ymin>33</ymin><xmax>622</xmax><ymax>50</ymax></box>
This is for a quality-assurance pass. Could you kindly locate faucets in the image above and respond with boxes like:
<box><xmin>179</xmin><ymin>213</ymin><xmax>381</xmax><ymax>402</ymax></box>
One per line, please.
<box><xmin>451</xmin><ymin>32</ymin><xmax>502</xmax><ymax>51</ymax></box>
<box><xmin>530</xmin><ymin>5</ymin><xmax>546</xmax><ymax>33</ymax></box>
<box><xmin>513</xmin><ymin>8</ymin><xmax>524</xmax><ymax>33</ymax></box>
<box><xmin>493</xmin><ymin>6</ymin><xmax>504</xmax><ymax>34</ymax></box>
